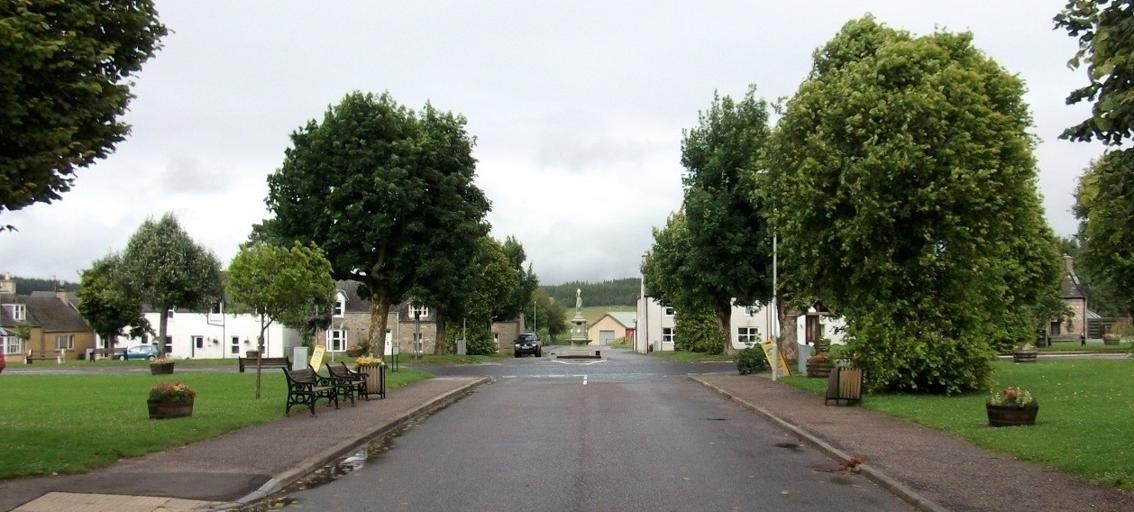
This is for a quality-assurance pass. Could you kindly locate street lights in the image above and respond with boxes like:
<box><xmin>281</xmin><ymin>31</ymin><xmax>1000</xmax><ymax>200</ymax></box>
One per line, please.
<box><xmin>757</xmin><ymin>169</ymin><xmax>777</xmax><ymax>382</ymax></box>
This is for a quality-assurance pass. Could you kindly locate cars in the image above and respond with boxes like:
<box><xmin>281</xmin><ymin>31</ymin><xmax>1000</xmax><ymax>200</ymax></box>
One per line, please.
<box><xmin>514</xmin><ymin>332</ymin><xmax>541</xmax><ymax>357</ymax></box>
<box><xmin>113</xmin><ymin>344</ymin><xmax>160</xmax><ymax>362</ymax></box>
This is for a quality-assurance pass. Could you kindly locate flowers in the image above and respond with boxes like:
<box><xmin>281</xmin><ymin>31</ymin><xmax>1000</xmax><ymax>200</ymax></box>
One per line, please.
<box><xmin>356</xmin><ymin>355</ymin><xmax>388</xmax><ymax>368</ymax></box>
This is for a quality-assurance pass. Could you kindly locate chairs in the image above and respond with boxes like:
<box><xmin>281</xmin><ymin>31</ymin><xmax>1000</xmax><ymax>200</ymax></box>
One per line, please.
<box><xmin>324</xmin><ymin>361</ymin><xmax>370</xmax><ymax>406</ymax></box>
<box><xmin>282</xmin><ymin>364</ymin><xmax>339</xmax><ymax>416</ymax></box>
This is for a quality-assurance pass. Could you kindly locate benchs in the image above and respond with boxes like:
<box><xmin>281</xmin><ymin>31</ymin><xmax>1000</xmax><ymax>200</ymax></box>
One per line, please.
<box><xmin>90</xmin><ymin>347</ymin><xmax>129</xmax><ymax>363</ymax></box>
<box><xmin>23</xmin><ymin>348</ymin><xmax>66</xmax><ymax>365</ymax></box>
<box><xmin>239</xmin><ymin>355</ymin><xmax>292</xmax><ymax>372</ymax></box>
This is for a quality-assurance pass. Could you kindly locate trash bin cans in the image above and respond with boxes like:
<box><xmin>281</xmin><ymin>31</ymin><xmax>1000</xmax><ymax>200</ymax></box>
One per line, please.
<box><xmin>1037</xmin><ymin>328</ymin><xmax>1048</xmax><ymax>348</ymax></box>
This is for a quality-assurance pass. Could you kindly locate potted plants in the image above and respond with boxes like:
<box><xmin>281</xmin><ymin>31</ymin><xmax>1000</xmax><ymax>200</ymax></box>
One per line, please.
<box><xmin>986</xmin><ymin>385</ymin><xmax>1039</xmax><ymax>425</ymax></box>
<box><xmin>147</xmin><ymin>383</ymin><xmax>197</xmax><ymax>419</ymax></box>
<box><xmin>150</xmin><ymin>354</ymin><xmax>175</xmax><ymax>374</ymax></box>
<box><xmin>806</xmin><ymin>353</ymin><xmax>834</xmax><ymax>378</ymax></box>
<box><xmin>1014</xmin><ymin>343</ymin><xmax>1038</xmax><ymax>362</ymax></box>
<box><xmin>814</xmin><ymin>336</ymin><xmax>831</xmax><ymax>355</ymax></box>
